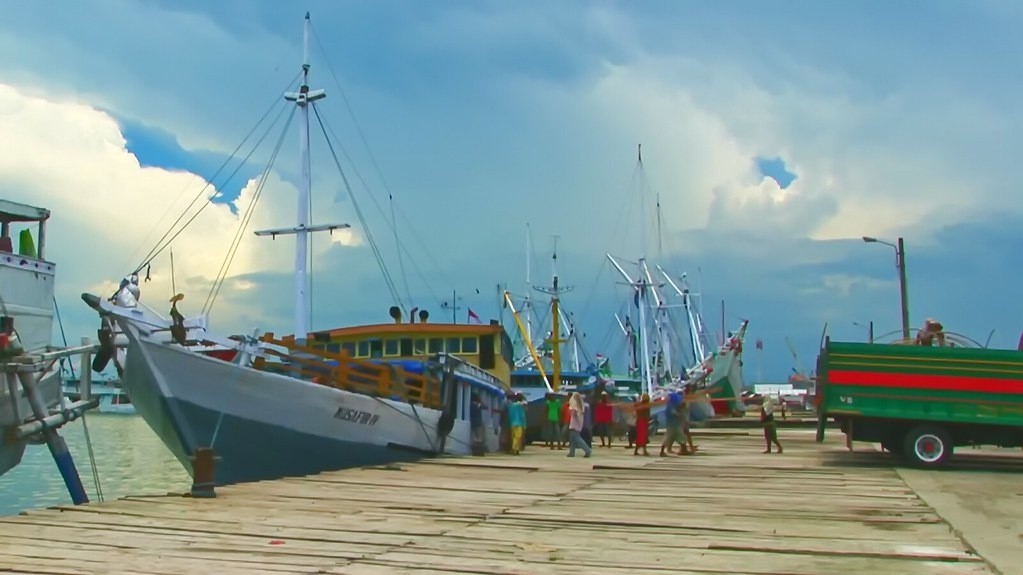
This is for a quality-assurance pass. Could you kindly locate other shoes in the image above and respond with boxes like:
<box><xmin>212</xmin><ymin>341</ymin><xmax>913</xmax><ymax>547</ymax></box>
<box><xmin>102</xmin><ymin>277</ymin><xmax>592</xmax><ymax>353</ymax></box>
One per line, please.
<box><xmin>643</xmin><ymin>453</ymin><xmax>650</xmax><ymax>457</ymax></box>
<box><xmin>632</xmin><ymin>451</ymin><xmax>639</xmax><ymax>456</ymax></box>
<box><xmin>566</xmin><ymin>454</ymin><xmax>575</xmax><ymax>458</ymax></box>
<box><xmin>583</xmin><ymin>453</ymin><xmax>590</xmax><ymax>457</ymax></box>
<box><xmin>660</xmin><ymin>453</ymin><xmax>667</xmax><ymax>457</ymax></box>
<box><xmin>667</xmin><ymin>450</ymin><xmax>675</xmax><ymax>454</ymax></box>
<box><xmin>690</xmin><ymin>444</ymin><xmax>699</xmax><ymax>451</ymax></box>
<box><xmin>778</xmin><ymin>447</ymin><xmax>783</xmax><ymax>454</ymax></box>
<box><xmin>763</xmin><ymin>449</ymin><xmax>771</xmax><ymax>454</ymax></box>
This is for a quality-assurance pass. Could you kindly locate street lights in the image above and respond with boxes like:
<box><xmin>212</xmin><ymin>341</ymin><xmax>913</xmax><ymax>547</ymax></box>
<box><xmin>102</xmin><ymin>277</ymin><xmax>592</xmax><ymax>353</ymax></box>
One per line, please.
<box><xmin>859</xmin><ymin>233</ymin><xmax>911</xmax><ymax>341</ymax></box>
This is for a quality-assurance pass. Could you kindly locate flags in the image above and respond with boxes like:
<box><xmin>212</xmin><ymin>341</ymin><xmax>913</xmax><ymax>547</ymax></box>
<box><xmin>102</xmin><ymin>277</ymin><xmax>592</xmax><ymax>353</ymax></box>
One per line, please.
<box><xmin>467</xmin><ymin>309</ymin><xmax>479</xmax><ymax>319</ymax></box>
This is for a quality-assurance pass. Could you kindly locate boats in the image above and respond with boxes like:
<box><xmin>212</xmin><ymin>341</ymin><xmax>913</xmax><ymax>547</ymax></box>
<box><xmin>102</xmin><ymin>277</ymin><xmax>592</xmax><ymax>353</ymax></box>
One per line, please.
<box><xmin>815</xmin><ymin>337</ymin><xmax>1020</xmax><ymax>470</ymax></box>
<box><xmin>2</xmin><ymin>201</ymin><xmax>86</xmax><ymax>476</ymax></box>
<box><xmin>75</xmin><ymin>12</ymin><xmax>504</xmax><ymax>488</ymax></box>
<box><xmin>60</xmin><ymin>348</ymin><xmax>136</xmax><ymax>416</ymax></box>
<box><xmin>297</xmin><ymin>316</ymin><xmax>751</xmax><ymax>442</ymax></box>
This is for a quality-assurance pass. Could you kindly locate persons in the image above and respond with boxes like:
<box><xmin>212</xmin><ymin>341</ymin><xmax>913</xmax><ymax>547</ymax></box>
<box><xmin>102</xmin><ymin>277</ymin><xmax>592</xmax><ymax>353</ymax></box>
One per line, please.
<box><xmin>915</xmin><ymin>318</ymin><xmax>946</xmax><ymax>346</ymax></box>
<box><xmin>468</xmin><ymin>393</ymin><xmax>490</xmax><ymax>456</ymax></box>
<box><xmin>497</xmin><ymin>388</ymin><xmax>530</xmax><ymax>455</ymax></box>
<box><xmin>623</xmin><ymin>396</ymin><xmax>639</xmax><ymax>449</ymax></box>
<box><xmin>761</xmin><ymin>395</ymin><xmax>783</xmax><ymax>453</ymax></box>
<box><xmin>595</xmin><ymin>391</ymin><xmax>614</xmax><ymax>448</ymax></box>
<box><xmin>659</xmin><ymin>392</ymin><xmax>699</xmax><ymax>456</ymax></box>
<box><xmin>781</xmin><ymin>399</ymin><xmax>787</xmax><ymax>421</ymax></box>
<box><xmin>633</xmin><ymin>393</ymin><xmax>650</xmax><ymax>456</ymax></box>
<box><xmin>566</xmin><ymin>391</ymin><xmax>592</xmax><ymax>457</ymax></box>
<box><xmin>543</xmin><ymin>391</ymin><xmax>574</xmax><ymax>450</ymax></box>
